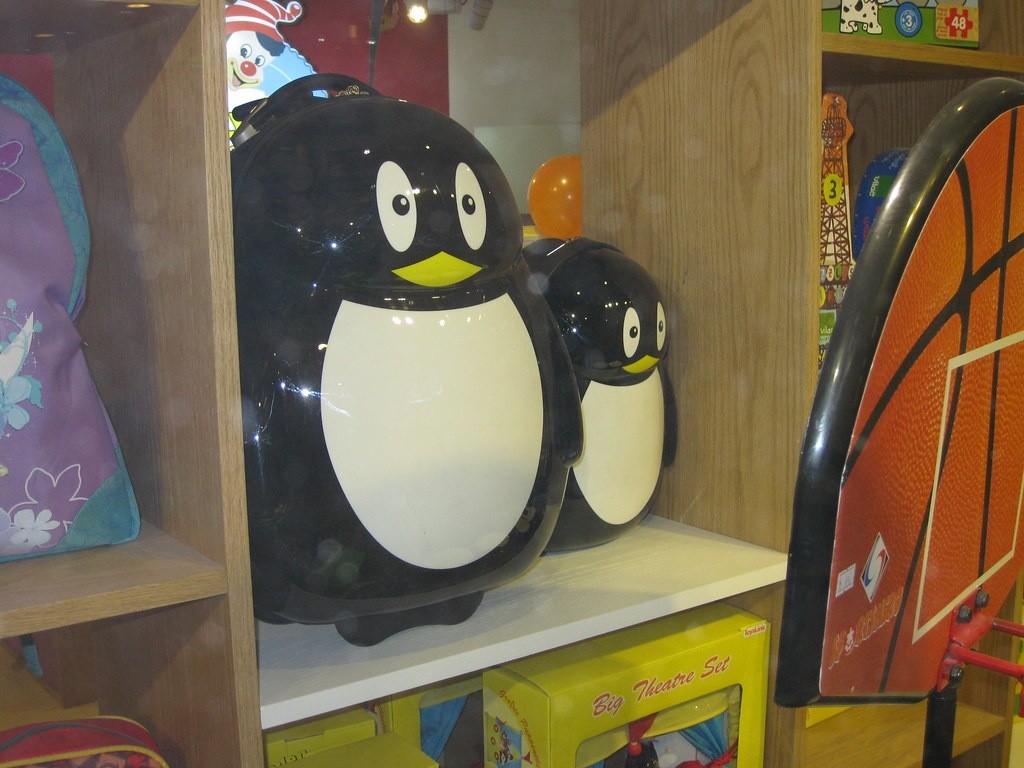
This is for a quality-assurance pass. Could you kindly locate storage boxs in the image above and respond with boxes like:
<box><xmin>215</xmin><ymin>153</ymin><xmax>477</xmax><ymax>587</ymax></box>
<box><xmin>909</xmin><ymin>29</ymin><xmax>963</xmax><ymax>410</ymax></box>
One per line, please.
<box><xmin>264</xmin><ymin>601</ymin><xmax>772</xmax><ymax>768</ymax></box>
<box><xmin>821</xmin><ymin>0</ymin><xmax>979</xmax><ymax>49</ymax></box>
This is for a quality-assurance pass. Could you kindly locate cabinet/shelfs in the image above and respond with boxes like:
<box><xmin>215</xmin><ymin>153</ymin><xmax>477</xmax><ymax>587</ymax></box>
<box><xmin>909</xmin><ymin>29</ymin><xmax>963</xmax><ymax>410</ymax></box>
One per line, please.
<box><xmin>0</xmin><ymin>0</ymin><xmax>1024</xmax><ymax>768</ymax></box>
